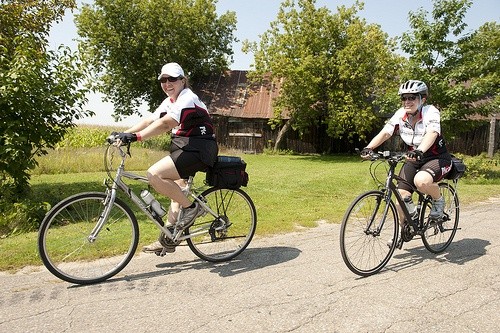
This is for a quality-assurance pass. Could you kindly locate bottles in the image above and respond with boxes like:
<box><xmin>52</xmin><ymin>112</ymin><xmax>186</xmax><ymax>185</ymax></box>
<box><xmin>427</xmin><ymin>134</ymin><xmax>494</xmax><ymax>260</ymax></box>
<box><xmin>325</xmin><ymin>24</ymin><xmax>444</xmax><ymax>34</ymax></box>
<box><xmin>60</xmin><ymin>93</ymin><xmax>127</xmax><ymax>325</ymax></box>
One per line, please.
<box><xmin>167</xmin><ymin>198</ymin><xmax>180</xmax><ymax>224</ymax></box>
<box><xmin>403</xmin><ymin>194</ymin><xmax>419</xmax><ymax>221</ymax></box>
<box><xmin>140</xmin><ymin>190</ymin><xmax>166</xmax><ymax>218</ymax></box>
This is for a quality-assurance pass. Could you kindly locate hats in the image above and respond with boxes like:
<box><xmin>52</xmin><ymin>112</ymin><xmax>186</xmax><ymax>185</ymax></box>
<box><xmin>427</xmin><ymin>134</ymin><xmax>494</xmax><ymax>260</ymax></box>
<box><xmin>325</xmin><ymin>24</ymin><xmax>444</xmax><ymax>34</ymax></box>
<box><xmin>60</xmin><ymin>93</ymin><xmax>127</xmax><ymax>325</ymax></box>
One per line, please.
<box><xmin>158</xmin><ymin>62</ymin><xmax>184</xmax><ymax>80</ymax></box>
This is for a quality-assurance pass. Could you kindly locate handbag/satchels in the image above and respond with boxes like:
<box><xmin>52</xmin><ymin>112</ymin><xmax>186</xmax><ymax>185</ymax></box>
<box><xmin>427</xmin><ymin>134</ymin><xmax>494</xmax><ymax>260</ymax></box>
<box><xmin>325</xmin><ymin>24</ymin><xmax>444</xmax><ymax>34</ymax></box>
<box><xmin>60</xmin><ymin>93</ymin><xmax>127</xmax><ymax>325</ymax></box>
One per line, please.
<box><xmin>206</xmin><ymin>155</ymin><xmax>249</xmax><ymax>190</ymax></box>
<box><xmin>444</xmin><ymin>153</ymin><xmax>466</xmax><ymax>180</ymax></box>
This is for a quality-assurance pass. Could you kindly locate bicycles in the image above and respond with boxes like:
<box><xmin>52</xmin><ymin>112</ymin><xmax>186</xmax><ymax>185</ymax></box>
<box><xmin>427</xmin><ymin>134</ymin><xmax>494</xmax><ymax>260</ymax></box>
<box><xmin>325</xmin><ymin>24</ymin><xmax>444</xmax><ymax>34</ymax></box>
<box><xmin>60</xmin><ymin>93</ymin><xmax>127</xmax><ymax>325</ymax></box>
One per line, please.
<box><xmin>38</xmin><ymin>135</ymin><xmax>257</xmax><ymax>284</ymax></box>
<box><xmin>340</xmin><ymin>148</ymin><xmax>461</xmax><ymax>276</ymax></box>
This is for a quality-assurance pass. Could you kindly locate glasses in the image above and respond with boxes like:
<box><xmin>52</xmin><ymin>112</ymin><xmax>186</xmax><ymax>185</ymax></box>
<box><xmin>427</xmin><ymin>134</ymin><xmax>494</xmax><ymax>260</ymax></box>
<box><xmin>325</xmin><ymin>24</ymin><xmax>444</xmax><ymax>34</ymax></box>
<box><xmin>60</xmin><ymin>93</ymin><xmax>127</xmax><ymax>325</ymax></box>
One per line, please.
<box><xmin>400</xmin><ymin>96</ymin><xmax>418</xmax><ymax>101</ymax></box>
<box><xmin>160</xmin><ymin>77</ymin><xmax>183</xmax><ymax>83</ymax></box>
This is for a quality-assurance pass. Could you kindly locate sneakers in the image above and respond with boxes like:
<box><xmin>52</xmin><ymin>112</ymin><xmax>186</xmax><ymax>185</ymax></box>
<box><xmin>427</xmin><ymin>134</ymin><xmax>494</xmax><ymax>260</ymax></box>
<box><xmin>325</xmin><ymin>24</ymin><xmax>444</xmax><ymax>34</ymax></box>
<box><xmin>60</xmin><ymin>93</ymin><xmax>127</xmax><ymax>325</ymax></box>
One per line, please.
<box><xmin>430</xmin><ymin>198</ymin><xmax>445</xmax><ymax>218</ymax></box>
<box><xmin>181</xmin><ymin>201</ymin><xmax>204</xmax><ymax>228</ymax></box>
<box><xmin>386</xmin><ymin>237</ymin><xmax>401</xmax><ymax>249</ymax></box>
<box><xmin>143</xmin><ymin>241</ymin><xmax>175</xmax><ymax>253</ymax></box>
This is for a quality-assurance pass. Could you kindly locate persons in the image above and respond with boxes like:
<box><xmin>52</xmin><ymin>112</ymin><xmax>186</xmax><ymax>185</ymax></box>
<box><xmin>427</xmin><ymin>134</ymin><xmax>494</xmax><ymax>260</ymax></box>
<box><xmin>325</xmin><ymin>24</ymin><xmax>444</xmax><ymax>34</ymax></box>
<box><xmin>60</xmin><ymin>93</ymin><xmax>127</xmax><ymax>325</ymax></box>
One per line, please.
<box><xmin>360</xmin><ymin>80</ymin><xmax>453</xmax><ymax>248</ymax></box>
<box><xmin>107</xmin><ymin>63</ymin><xmax>219</xmax><ymax>254</ymax></box>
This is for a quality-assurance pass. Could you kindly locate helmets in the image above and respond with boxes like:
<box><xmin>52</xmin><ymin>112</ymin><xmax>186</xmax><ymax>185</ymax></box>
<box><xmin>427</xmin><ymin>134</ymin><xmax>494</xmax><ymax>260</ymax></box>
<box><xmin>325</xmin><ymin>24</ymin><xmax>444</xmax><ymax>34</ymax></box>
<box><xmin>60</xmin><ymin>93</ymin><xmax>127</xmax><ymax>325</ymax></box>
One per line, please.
<box><xmin>398</xmin><ymin>79</ymin><xmax>428</xmax><ymax>97</ymax></box>
